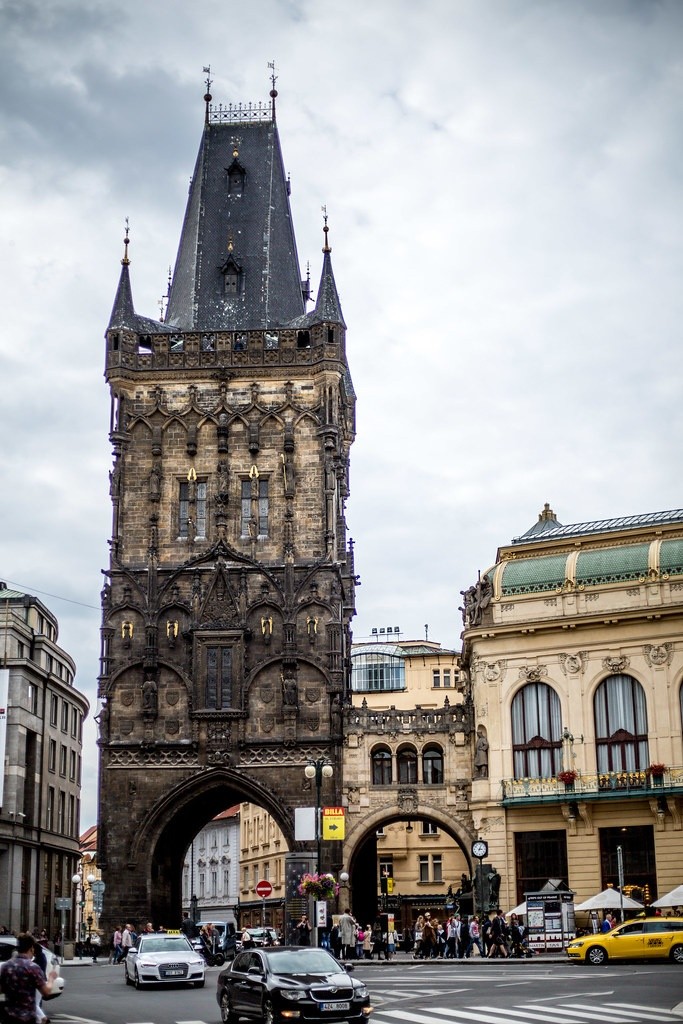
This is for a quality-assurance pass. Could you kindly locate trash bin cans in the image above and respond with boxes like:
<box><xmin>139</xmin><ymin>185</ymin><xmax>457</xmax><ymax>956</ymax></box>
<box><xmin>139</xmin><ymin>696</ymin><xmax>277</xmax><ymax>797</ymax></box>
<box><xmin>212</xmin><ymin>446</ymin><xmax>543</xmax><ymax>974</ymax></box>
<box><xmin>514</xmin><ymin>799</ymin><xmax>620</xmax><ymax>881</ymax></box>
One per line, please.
<box><xmin>64</xmin><ymin>941</ymin><xmax>73</xmax><ymax>960</ymax></box>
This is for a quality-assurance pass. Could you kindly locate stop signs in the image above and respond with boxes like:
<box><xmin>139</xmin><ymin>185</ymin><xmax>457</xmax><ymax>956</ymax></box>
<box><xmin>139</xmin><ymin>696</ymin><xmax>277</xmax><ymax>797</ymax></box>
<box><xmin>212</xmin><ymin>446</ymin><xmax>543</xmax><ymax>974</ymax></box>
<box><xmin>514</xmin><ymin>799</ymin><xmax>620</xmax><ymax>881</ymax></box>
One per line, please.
<box><xmin>256</xmin><ymin>880</ymin><xmax>272</xmax><ymax>898</ymax></box>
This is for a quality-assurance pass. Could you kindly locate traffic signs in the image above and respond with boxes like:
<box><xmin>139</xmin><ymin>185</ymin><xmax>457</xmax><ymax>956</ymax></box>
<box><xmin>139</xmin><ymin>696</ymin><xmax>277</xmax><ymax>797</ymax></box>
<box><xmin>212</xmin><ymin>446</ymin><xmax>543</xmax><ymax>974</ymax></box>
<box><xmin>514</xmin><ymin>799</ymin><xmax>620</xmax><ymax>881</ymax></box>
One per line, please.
<box><xmin>323</xmin><ymin>807</ymin><xmax>345</xmax><ymax>841</ymax></box>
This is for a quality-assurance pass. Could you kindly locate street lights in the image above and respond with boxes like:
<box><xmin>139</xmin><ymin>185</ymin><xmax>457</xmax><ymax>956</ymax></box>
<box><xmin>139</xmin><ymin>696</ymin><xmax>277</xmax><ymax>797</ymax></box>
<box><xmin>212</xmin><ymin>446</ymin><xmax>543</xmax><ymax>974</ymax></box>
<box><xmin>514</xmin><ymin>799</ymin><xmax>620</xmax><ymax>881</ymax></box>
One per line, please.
<box><xmin>86</xmin><ymin>915</ymin><xmax>94</xmax><ymax>956</ymax></box>
<box><xmin>71</xmin><ymin>864</ymin><xmax>97</xmax><ymax>962</ymax></box>
<box><xmin>302</xmin><ymin>757</ymin><xmax>352</xmax><ymax>946</ymax></box>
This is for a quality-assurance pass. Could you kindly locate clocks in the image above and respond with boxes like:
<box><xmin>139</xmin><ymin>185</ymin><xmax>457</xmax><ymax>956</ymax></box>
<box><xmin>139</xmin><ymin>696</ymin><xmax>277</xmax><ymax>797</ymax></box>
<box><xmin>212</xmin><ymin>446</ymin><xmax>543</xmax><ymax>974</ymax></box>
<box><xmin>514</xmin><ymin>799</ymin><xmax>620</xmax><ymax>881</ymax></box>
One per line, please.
<box><xmin>470</xmin><ymin>838</ymin><xmax>490</xmax><ymax>860</ymax></box>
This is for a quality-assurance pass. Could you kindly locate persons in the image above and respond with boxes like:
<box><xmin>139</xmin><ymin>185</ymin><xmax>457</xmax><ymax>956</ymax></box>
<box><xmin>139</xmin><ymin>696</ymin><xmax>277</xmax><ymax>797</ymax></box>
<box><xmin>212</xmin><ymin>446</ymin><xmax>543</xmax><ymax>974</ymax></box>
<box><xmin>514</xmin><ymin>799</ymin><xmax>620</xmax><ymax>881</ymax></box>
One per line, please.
<box><xmin>0</xmin><ymin>908</ymin><xmax>681</xmax><ymax>1024</ymax></box>
<box><xmin>471</xmin><ymin>730</ymin><xmax>489</xmax><ymax>779</ymax></box>
<box><xmin>458</xmin><ymin>575</ymin><xmax>494</xmax><ymax>624</ymax></box>
<box><xmin>141</xmin><ymin>673</ymin><xmax>158</xmax><ymax>709</ymax></box>
<box><xmin>283</xmin><ymin>671</ymin><xmax>297</xmax><ymax>705</ymax></box>
<box><xmin>94</xmin><ymin>702</ymin><xmax>109</xmax><ymax>739</ymax></box>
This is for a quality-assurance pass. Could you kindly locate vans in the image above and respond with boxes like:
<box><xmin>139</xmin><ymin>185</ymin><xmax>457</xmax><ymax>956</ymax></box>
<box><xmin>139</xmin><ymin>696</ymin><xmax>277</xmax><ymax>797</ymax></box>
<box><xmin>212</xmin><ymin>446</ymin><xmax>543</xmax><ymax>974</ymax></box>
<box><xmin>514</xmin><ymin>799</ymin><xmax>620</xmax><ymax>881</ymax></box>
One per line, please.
<box><xmin>191</xmin><ymin>921</ymin><xmax>236</xmax><ymax>966</ymax></box>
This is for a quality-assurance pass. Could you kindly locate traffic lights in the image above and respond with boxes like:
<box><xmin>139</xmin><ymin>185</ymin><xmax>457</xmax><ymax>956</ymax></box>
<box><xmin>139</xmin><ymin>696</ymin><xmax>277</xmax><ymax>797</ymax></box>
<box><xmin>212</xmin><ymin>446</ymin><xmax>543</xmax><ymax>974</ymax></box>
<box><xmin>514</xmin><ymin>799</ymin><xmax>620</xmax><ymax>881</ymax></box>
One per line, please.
<box><xmin>608</xmin><ymin>883</ymin><xmax>614</xmax><ymax>888</ymax></box>
<box><xmin>625</xmin><ymin>886</ymin><xmax>631</xmax><ymax>898</ymax></box>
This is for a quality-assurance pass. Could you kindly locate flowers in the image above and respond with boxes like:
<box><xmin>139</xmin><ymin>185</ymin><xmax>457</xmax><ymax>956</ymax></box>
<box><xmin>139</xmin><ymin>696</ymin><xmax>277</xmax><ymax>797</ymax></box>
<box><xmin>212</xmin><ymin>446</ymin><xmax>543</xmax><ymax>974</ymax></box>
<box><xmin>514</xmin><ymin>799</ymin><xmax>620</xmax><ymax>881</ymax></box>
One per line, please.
<box><xmin>643</xmin><ymin>762</ymin><xmax>669</xmax><ymax>775</ymax></box>
<box><xmin>297</xmin><ymin>870</ymin><xmax>340</xmax><ymax>900</ymax></box>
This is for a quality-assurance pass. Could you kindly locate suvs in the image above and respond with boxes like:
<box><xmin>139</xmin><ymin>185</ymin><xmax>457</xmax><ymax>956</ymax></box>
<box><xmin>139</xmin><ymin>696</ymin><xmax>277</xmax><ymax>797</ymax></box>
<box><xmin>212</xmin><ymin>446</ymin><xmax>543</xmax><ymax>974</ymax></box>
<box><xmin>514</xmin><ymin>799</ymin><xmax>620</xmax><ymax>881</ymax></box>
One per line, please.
<box><xmin>0</xmin><ymin>935</ymin><xmax>65</xmax><ymax>1006</ymax></box>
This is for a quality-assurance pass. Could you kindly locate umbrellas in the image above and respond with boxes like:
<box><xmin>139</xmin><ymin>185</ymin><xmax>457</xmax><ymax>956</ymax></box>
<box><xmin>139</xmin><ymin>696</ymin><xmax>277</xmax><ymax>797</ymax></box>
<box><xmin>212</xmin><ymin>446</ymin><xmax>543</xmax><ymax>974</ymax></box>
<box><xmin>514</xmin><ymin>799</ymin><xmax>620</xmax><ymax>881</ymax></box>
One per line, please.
<box><xmin>650</xmin><ymin>885</ymin><xmax>683</xmax><ymax>907</ymax></box>
<box><xmin>506</xmin><ymin>901</ymin><xmax>526</xmax><ymax>916</ymax></box>
<box><xmin>574</xmin><ymin>887</ymin><xmax>645</xmax><ymax>912</ymax></box>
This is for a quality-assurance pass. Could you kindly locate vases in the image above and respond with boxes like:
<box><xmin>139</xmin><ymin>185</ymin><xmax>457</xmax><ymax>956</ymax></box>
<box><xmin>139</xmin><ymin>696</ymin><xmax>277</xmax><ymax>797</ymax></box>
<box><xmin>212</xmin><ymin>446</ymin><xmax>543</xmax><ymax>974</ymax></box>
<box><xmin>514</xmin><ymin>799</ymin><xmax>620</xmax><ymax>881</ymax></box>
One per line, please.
<box><xmin>565</xmin><ymin>780</ymin><xmax>576</xmax><ymax>792</ymax></box>
<box><xmin>653</xmin><ymin>773</ymin><xmax>665</xmax><ymax>787</ymax></box>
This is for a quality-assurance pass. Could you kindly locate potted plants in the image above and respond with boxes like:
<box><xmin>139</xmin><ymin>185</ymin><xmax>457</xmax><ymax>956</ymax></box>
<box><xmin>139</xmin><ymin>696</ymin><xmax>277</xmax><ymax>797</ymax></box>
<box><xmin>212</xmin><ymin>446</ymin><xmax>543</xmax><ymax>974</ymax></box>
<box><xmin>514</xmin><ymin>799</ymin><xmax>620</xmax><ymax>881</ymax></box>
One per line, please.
<box><xmin>557</xmin><ymin>767</ymin><xmax>578</xmax><ymax>780</ymax></box>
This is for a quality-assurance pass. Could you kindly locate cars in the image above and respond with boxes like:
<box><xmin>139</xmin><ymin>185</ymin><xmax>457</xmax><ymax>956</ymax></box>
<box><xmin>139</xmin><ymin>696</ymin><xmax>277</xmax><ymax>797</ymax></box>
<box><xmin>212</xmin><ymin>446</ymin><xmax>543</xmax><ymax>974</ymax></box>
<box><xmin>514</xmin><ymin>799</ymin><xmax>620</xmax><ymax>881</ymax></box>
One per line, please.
<box><xmin>566</xmin><ymin>916</ymin><xmax>683</xmax><ymax>964</ymax></box>
<box><xmin>215</xmin><ymin>945</ymin><xmax>371</xmax><ymax>1024</ymax></box>
<box><xmin>235</xmin><ymin>926</ymin><xmax>280</xmax><ymax>953</ymax></box>
<box><xmin>124</xmin><ymin>930</ymin><xmax>205</xmax><ymax>990</ymax></box>
